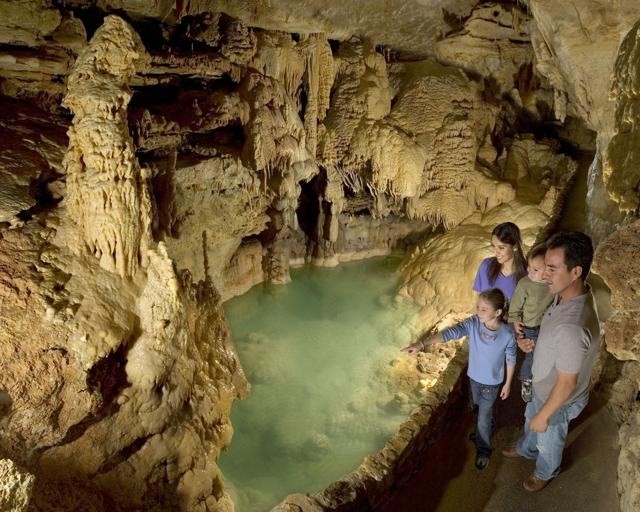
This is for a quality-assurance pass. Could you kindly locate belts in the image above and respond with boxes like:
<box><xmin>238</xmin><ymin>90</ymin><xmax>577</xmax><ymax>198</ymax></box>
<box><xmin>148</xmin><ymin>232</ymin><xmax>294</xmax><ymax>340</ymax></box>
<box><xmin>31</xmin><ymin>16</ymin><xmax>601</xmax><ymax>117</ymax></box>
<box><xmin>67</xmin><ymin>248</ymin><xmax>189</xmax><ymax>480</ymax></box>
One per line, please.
<box><xmin>523</xmin><ymin>326</ymin><xmax>539</xmax><ymax>330</ymax></box>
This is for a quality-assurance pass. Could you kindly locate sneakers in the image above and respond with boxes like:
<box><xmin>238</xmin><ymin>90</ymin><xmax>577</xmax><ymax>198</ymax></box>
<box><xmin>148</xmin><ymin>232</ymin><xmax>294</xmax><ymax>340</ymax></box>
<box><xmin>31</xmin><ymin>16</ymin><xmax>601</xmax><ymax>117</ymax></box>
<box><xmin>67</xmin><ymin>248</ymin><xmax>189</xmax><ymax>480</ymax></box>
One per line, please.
<box><xmin>468</xmin><ymin>423</ymin><xmax>496</xmax><ymax>441</ymax></box>
<box><xmin>522</xmin><ymin>472</ymin><xmax>555</xmax><ymax>492</ymax></box>
<box><xmin>521</xmin><ymin>378</ymin><xmax>532</xmax><ymax>403</ymax></box>
<box><xmin>500</xmin><ymin>446</ymin><xmax>522</xmax><ymax>458</ymax></box>
<box><xmin>475</xmin><ymin>454</ymin><xmax>490</xmax><ymax>469</ymax></box>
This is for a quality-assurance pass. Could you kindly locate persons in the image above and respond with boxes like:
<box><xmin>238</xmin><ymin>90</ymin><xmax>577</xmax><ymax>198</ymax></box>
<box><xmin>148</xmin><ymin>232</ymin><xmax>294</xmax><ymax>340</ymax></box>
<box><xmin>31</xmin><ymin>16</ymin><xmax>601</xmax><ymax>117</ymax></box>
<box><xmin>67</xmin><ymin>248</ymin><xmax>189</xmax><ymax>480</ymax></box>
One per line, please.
<box><xmin>507</xmin><ymin>241</ymin><xmax>553</xmax><ymax>404</ymax></box>
<box><xmin>469</xmin><ymin>222</ymin><xmax>529</xmax><ymax>312</ymax></box>
<box><xmin>500</xmin><ymin>228</ymin><xmax>603</xmax><ymax>493</ymax></box>
<box><xmin>399</xmin><ymin>286</ymin><xmax>519</xmax><ymax>470</ymax></box>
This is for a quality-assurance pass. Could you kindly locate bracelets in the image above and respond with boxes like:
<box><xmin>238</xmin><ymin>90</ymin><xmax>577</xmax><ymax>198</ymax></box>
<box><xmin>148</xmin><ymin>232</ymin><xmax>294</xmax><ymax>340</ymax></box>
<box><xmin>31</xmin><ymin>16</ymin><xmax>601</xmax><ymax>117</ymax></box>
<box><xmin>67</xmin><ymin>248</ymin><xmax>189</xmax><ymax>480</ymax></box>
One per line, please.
<box><xmin>419</xmin><ymin>340</ymin><xmax>425</xmax><ymax>350</ymax></box>
